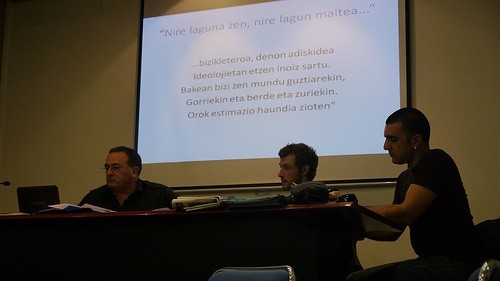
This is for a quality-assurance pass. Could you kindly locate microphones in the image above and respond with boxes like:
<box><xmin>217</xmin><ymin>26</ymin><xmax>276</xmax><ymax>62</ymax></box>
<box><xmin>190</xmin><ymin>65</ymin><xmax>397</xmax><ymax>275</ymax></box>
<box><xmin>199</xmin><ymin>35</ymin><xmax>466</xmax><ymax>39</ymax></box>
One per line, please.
<box><xmin>0</xmin><ymin>180</ymin><xmax>10</xmax><ymax>186</ymax></box>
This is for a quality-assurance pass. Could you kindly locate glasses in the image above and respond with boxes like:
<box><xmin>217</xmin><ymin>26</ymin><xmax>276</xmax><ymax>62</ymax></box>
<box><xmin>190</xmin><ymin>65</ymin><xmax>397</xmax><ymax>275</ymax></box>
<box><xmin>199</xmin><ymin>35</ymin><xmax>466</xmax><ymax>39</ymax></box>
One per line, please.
<box><xmin>101</xmin><ymin>165</ymin><xmax>134</xmax><ymax>172</ymax></box>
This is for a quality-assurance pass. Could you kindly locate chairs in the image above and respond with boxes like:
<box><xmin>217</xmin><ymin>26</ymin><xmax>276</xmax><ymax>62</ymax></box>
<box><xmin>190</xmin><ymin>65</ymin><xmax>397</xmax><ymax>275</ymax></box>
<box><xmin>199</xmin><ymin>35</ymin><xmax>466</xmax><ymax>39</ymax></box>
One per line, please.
<box><xmin>207</xmin><ymin>264</ymin><xmax>297</xmax><ymax>281</ymax></box>
<box><xmin>467</xmin><ymin>258</ymin><xmax>500</xmax><ymax>281</ymax></box>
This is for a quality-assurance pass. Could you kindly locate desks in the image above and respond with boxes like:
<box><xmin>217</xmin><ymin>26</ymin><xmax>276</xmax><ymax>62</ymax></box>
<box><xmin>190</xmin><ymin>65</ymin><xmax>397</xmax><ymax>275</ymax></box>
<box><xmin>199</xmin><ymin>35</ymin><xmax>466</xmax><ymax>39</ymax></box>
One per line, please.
<box><xmin>0</xmin><ymin>200</ymin><xmax>359</xmax><ymax>281</ymax></box>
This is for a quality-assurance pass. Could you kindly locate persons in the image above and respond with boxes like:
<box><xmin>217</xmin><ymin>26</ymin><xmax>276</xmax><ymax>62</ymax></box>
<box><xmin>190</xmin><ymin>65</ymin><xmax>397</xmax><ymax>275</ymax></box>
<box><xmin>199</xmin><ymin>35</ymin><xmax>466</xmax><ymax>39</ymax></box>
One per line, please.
<box><xmin>77</xmin><ymin>145</ymin><xmax>184</xmax><ymax>212</ymax></box>
<box><xmin>330</xmin><ymin>105</ymin><xmax>483</xmax><ymax>281</ymax></box>
<box><xmin>277</xmin><ymin>141</ymin><xmax>319</xmax><ymax>191</ymax></box>
